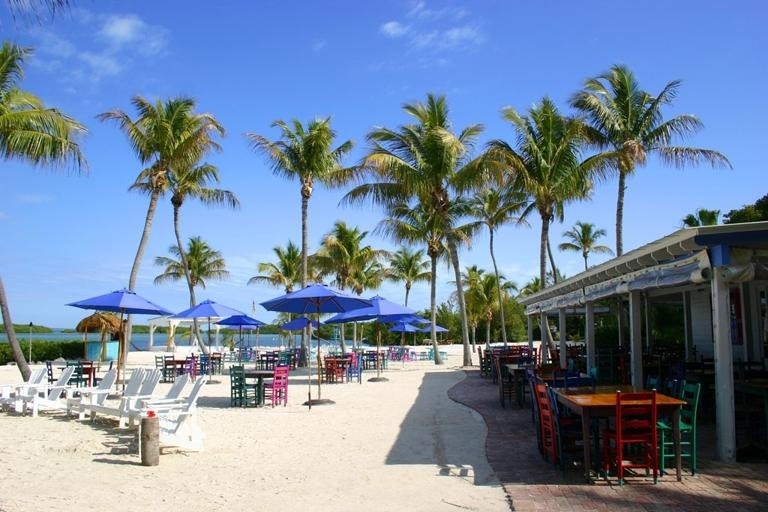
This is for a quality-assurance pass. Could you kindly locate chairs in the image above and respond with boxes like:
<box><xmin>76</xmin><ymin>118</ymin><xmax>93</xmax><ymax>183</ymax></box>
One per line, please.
<box><xmin>478</xmin><ymin>341</ymin><xmax>704</xmax><ymax>486</ymax></box>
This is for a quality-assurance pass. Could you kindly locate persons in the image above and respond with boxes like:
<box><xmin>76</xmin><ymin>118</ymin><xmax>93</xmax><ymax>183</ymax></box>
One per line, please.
<box><xmin>653</xmin><ymin>335</ymin><xmax>663</xmax><ymax>351</ymax></box>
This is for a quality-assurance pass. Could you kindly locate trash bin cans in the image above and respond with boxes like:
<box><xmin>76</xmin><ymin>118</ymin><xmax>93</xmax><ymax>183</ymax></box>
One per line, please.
<box><xmin>297</xmin><ymin>348</ymin><xmax>307</xmax><ymax>367</ymax></box>
